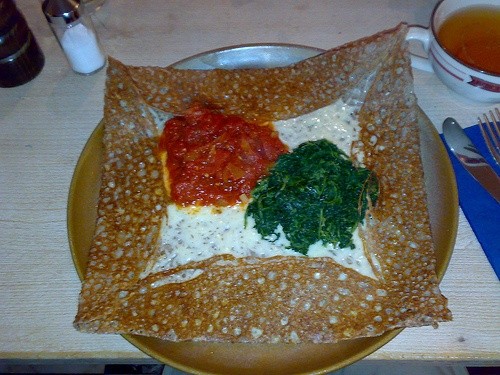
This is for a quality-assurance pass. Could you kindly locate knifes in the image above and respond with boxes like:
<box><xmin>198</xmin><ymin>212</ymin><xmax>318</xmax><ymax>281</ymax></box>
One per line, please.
<box><xmin>443</xmin><ymin>117</ymin><xmax>500</xmax><ymax>204</ymax></box>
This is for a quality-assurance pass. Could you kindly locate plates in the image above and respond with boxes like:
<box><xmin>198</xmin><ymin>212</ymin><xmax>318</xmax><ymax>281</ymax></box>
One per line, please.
<box><xmin>67</xmin><ymin>43</ymin><xmax>459</xmax><ymax>375</ymax></box>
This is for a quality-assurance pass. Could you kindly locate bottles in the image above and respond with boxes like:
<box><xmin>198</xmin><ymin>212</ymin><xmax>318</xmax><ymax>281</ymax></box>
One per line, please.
<box><xmin>43</xmin><ymin>0</ymin><xmax>107</xmax><ymax>77</ymax></box>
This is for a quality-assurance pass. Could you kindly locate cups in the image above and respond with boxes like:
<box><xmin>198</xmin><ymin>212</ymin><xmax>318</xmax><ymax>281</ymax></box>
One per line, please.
<box><xmin>0</xmin><ymin>0</ymin><xmax>45</xmax><ymax>87</ymax></box>
<box><xmin>404</xmin><ymin>0</ymin><xmax>500</xmax><ymax>105</ymax></box>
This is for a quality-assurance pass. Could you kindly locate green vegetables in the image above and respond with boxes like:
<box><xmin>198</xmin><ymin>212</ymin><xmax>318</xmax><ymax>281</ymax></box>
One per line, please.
<box><xmin>243</xmin><ymin>138</ymin><xmax>381</xmax><ymax>257</ymax></box>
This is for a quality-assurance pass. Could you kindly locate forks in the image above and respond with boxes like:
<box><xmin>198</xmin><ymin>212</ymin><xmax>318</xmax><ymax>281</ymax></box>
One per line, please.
<box><xmin>478</xmin><ymin>107</ymin><xmax>500</xmax><ymax>165</ymax></box>
